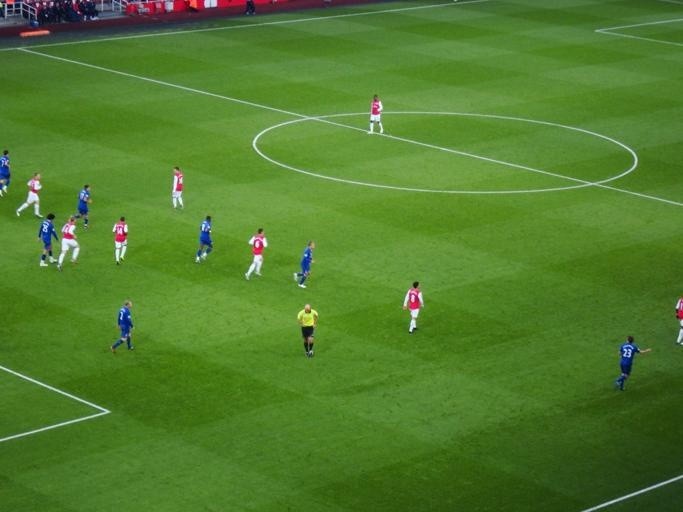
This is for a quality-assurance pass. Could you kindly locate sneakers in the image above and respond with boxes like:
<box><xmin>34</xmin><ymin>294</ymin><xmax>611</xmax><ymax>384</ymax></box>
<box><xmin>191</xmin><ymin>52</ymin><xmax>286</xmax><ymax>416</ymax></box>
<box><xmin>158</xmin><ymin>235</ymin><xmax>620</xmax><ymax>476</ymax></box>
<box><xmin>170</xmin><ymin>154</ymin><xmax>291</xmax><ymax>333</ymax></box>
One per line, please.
<box><xmin>409</xmin><ymin>328</ymin><xmax>417</xmax><ymax>333</ymax></box>
<box><xmin>115</xmin><ymin>256</ymin><xmax>124</xmax><ymax>265</ymax></box>
<box><xmin>0</xmin><ymin>186</ymin><xmax>41</xmax><ymax>218</ymax></box>
<box><xmin>196</xmin><ymin>255</ymin><xmax>206</xmax><ymax>263</ymax></box>
<box><xmin>294</xmin><ymin>273</ymin><xmax>298</xmax><ymax>281</ymax></box>
<box><xmin>368</xmin><ymin>129</ymin><xmax>383</xmax><ymax>134</ymax></box>
<box><xmin>298</xmin><ymin>284</ymin><xmax>306</xmax><ymax>289</ymax></box>
<box><xmin>40</xmin><ymin>257</ymin><xmax>78</xmax><ymax>271</ymax></box>
<box><xmin>110</xmin><ymin>345</ymin><xmax>133</xmax><ymax>353</ymax></box>
<box><xmin>245</xmin><ymin>273</ymin><xmax>249</xmax><ymax>281</ymax></box>
<box><xmin>255</xmin><ymin>272</ymin><xmax>261</xmax><ymax>276</ymax></box>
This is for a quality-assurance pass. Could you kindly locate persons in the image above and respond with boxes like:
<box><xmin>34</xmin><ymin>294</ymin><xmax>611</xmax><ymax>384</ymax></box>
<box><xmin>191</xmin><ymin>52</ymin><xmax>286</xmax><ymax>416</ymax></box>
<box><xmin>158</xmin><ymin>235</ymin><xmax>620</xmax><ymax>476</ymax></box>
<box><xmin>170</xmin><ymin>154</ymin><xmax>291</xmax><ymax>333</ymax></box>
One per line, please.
<box><xmin>110</xmin><ymin>300</ymin><xmax>135</xmax><ymax>352</ymax></box>
<box><xmin>16</xmin><ymin>173</ymin><xmax>44</xmax><ymax>218</ymax></box>
<box><xmin>675</xmin><ymin>298</ymin><xmax>683</xmax><ymax>345</ymax></box>
<box><xmin>293</xmin><ymin>240</ymin><xmax>315</xmax><ymax>288</ymax></box>
<box><xmin>21</xmin><ymin>0</ymin><xmax>99</xmax><ymax>26</ymax></box>
<box><xmin>402</xmin><ymin>281</ymin><xmax>425</xmax><ymax>335</ymax></box>
<box><xmin>245</xmin><ymin>0</ymin><xmax>256</xmax><ymax>16</ymax></box>
<box><xmin>297</xmin><ymin>304</ymin><xmax>318</xmax><ymax>359</ymax></box>
<box><xmin>615</xmin><ymin>335</ymin><xmax>652</xmax><ymax>392</ymax></box>
<box><xmin>194</xmin><ymin>215</ymin><xmax>213</xmax><ymax>263</ymax></box>
<box><xmin>57</xmin><ymin>216</ymin><xmax>80</xmax><ymax>272</ymax></box>
<box><xmin>0</xmin><ymin>149</ymin><xmax>11</xmax><ymax>197</ymax></box>
<box><xmin>70</xmin><ymin>184</ymin><xmax>92</xmax><ymax>230</ymax></box>
<box><xmin>244</xmin><ymin>228</ymin><xmax>268</xmax><ymax>281</ymax></box>
<box><xmin>171</xmin><ymin>166</ymin><xmax>184</xmax><ymax>209</ymax></box>
<box><xmin>38</xmin><ymin>213</ymin><xmax>59</xmax><ymax>267</ymax></box>
<box><xmin>113</xmin><ymin>216</ymin><xmax>129</xmax><ymax>265</ymax></box>
<box><xmin>369</xmin><ymin>94</ymin><xmax>383</xmax><ymax>135</ymax></box>
